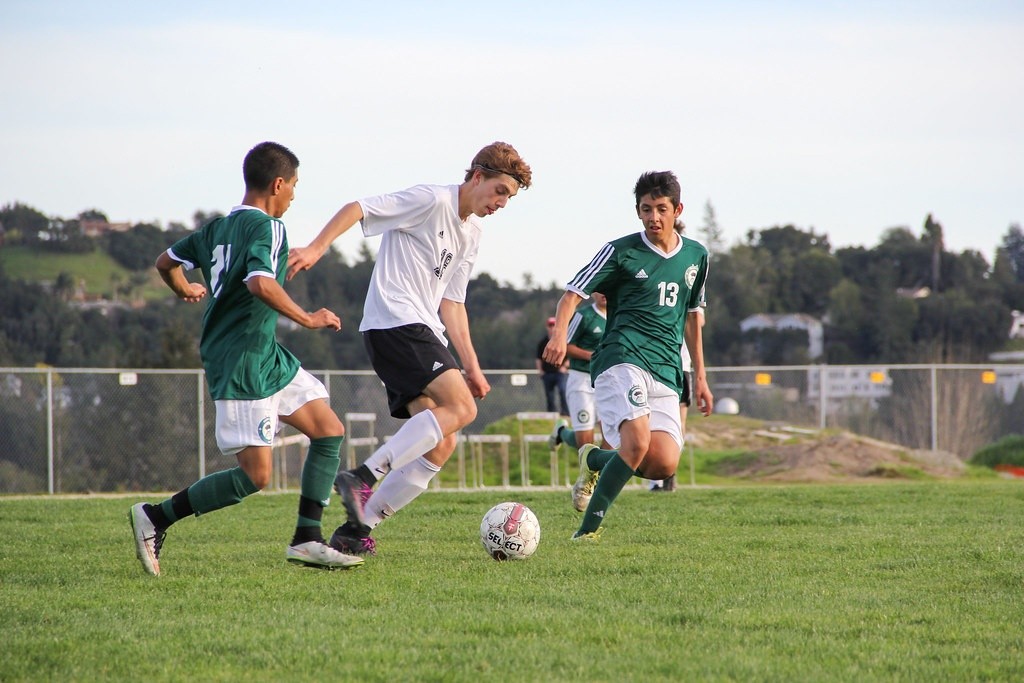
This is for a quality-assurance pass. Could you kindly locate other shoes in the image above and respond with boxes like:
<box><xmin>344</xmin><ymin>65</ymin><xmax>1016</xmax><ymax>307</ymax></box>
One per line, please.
<box><xmin>650</xmin><ymin>484</ymin><xmax>663</xmax><ymax>490</ymax></box>
<box><xmin>663</xmin><ymin>473</ymin><xmax>677</xmax><ymax>492</ymax></box>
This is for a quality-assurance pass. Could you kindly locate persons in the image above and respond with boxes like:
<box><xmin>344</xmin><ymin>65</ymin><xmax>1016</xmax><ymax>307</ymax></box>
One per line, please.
<box><xmin>542</xmin><ymin>172</ymin><xmax>713</xmax><ymax>540</ymax></box>
<box><xmin>537</xmin><ymin>223</ymin><xmax>707</xmax><ymax>491</ymax></box>
<box><xmin>286</xmin><ymin>141</ymin><xmax>533</xmax><ymax>556</ymax></box>
<box><xmin>129</xmin><ymin>141</ymin><xmax>363</xmax><ymax>578</ymax></box>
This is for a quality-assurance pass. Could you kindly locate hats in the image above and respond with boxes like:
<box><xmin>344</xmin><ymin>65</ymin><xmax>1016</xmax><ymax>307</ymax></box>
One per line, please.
<box><xmin>546</xmin><ymin>317</ymin><xmax>556</xmax><ymax>325</ymax></box>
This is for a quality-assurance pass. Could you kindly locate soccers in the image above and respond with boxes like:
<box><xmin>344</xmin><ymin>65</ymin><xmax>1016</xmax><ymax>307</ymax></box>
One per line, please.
<box><xmin>479</xmin><ymin>501</ymin><xmax>541</xmax><ymax>561</ymax></box>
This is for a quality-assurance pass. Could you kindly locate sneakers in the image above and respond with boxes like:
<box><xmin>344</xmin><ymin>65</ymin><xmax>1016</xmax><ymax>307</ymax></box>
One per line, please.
<box><xmin>335</xmin><ymin>471</ymin><xmax>374</xmax><ymax>529</ymax></box>
<box><xmin>571</xmin><ymin>527</ymin><xmax>605</xmax><ymax>544</ymax></box>
<box><xmin>286</xmin><ymin>536</ymin><xmax>365</xmax><ymax>570</ymax></box>
<box><xmin>128</xmin><ymin>502</ymin><xmax>167</xmax><ymax>577</ymax></box>
<box><xmin>329</xmin><ymin>527</ymin><xmax>377</xmax><ymax>557</ymax></box>
<box><xmin>572</xmin><ymin>443</ymin><xmax>601</xmax><ymax>512</ymax></box>
<box><xmin>549</xmin><ymin>418</ymin><xmax>568</xmax><ymax>451</ymax></box>
